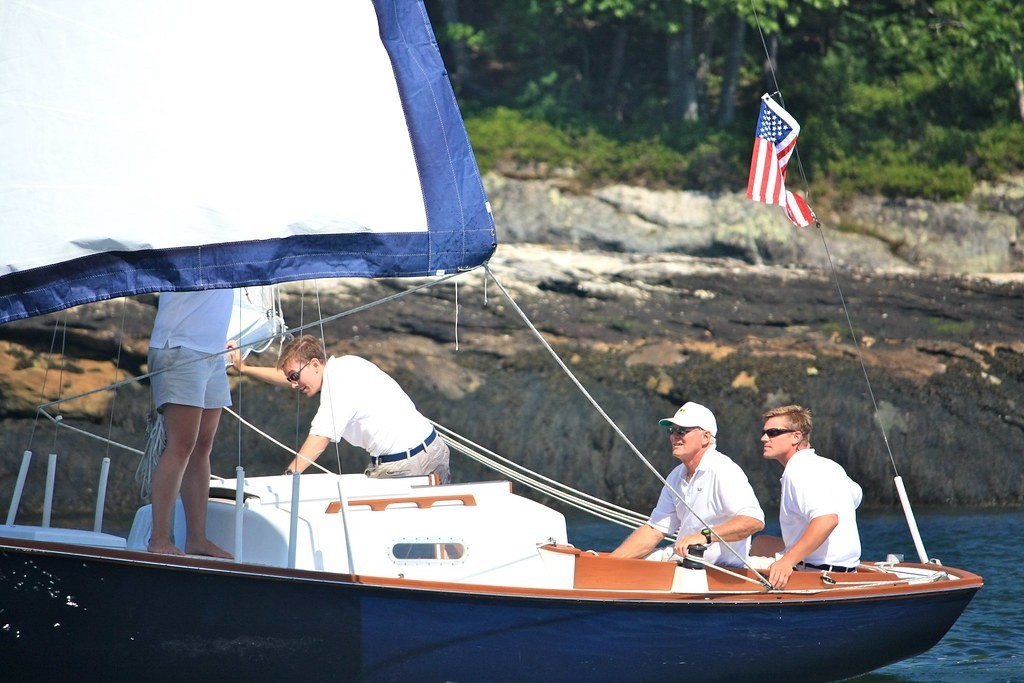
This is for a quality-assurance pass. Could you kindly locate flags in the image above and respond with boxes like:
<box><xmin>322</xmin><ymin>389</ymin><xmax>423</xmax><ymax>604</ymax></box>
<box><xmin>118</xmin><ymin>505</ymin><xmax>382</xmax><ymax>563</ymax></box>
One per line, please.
<box><xmin>747</xmin><ymin>93</ymin><xmax>817</xmax><ymax>226</ymax></box>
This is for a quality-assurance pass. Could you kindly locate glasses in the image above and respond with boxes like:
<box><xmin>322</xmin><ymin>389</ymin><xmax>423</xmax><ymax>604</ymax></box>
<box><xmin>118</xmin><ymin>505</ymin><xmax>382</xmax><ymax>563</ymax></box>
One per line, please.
<box><xmin>666</xmin><ymin>426</ymin><xmax>690</xmax><ymax>434</ymax></box>
<box><xmin>287</xmin><ymin>361</ymin><xmax>310</xmax><ymax>382</ymax></box>
<box><xmin>762</xmin><ymin>428</ymin><xmax>795</xmax><ymax>437</ymax></box>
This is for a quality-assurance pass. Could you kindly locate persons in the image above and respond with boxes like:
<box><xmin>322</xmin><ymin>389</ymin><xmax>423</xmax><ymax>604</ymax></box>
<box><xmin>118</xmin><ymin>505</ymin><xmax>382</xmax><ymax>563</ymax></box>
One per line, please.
<box><xmin>758</xmin><ymin>405</ymin><xmax>863</xmax><ymax>591</ymax></box>
<box><xmin>609</xmin><ymin>401</ymin><xmax>764</xmax><ymax>570</ymax></box>
<box><xmin>226</xmin><ymin>334</ymin><xmax>451</xmax><ymax>488</ymax></box>
<box><xmin>145</xmin><ymin>288</ymin><xmax>236</xmax><ymax>561</ymax></box>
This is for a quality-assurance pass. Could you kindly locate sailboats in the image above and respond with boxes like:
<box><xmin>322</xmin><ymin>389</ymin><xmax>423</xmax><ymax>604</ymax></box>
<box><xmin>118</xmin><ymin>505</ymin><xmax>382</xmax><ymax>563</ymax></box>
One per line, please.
<box><xmin>0</xmin><ymin>0</ymin><xmax>984</xmax><ymax>683</ymax></box>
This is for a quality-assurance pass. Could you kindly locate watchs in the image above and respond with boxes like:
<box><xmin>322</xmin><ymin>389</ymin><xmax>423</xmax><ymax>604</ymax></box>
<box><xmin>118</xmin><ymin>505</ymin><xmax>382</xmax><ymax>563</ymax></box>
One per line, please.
<box><xmin>701</xmin><ymin>529</ymin><xmax>712</xmax><ymax>544</ymax></box>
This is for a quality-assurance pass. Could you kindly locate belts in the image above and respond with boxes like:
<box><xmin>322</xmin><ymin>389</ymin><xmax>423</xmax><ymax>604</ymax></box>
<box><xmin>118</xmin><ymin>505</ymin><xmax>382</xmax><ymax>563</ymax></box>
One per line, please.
<box><xmin>371</xmin><ymin>426</ymin><xmax>436</xmax><ymax>465</ymax></box>
<box><xmin>798</xmin><ymin>560</ymin><xmax>855</xmax><ymax>572</ymax></box>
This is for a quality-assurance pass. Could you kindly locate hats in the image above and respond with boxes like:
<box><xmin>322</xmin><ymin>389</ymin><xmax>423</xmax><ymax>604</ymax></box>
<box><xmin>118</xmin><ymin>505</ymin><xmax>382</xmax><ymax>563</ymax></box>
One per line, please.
<box><xmin>659</xmin><ymin>401</ymin><xmax>717</xmax><ymax>436</ymax></box>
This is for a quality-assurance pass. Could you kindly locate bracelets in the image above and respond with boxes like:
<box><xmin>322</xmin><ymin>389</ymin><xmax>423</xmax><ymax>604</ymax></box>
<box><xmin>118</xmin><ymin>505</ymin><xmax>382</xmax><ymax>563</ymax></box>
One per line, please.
<box><xmin>283</xmin><ymin>468</ymin><xmax>293</xmax><ymax>475</ymax></box>
